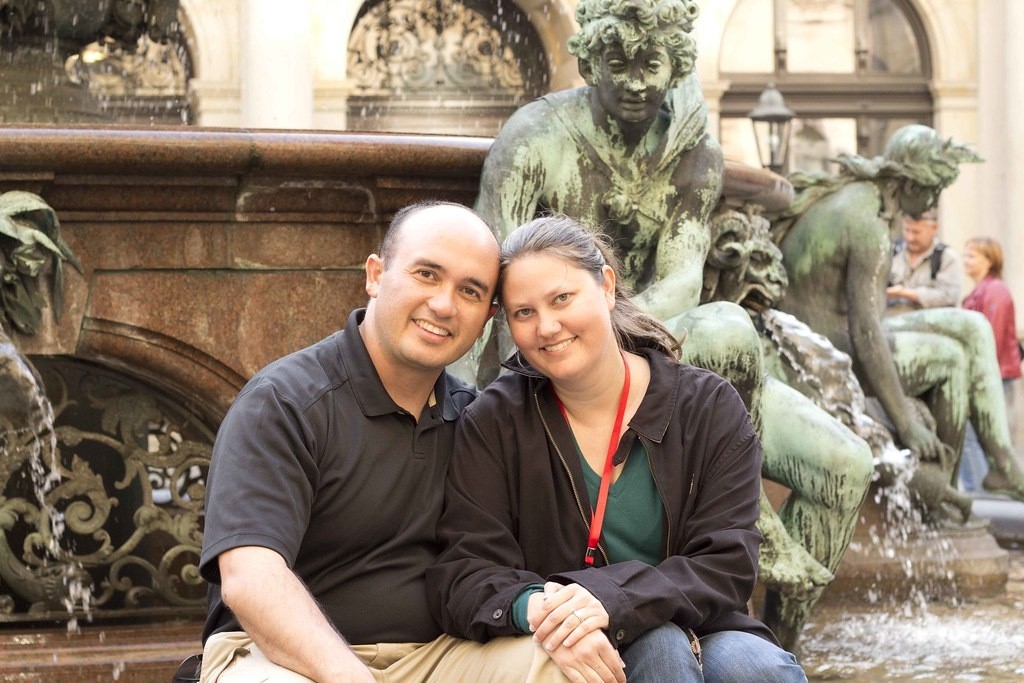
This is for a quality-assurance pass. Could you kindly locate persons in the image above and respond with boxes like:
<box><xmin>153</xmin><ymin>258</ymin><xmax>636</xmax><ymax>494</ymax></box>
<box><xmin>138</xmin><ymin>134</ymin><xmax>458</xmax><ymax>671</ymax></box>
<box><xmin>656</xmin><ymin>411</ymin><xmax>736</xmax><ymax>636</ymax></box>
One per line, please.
<box><xmin>473</xmin><ymin>1</ymin><xmax>1024</xmax><ymax>650</ymax></box>
<box><xmin>960</xmin><ymin>237</ymin><xmax>1023</xmax><ymax>492</ymax></box>
<box><xmin>886</xmin><ymin>200</ymin><xmax>960</xmax><ymax>311</ymax></box>
<box><xmin>201</xmin><ymin>202</ymin><xmax>570</xmax><ymax>683</ymax></box>
<box><xmin>422</xmin><ymin>216</ymin><xmax>812</xmax><ymax>683</ymax></box>
